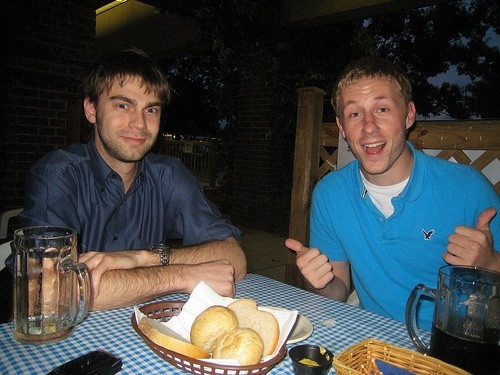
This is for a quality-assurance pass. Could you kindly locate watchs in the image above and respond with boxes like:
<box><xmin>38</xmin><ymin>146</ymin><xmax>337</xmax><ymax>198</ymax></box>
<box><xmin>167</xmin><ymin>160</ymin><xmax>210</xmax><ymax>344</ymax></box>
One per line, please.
<box><xmin>151</xmin><ymin>244</ymin><xmax>170</xmax><ymax>265</ymax></box>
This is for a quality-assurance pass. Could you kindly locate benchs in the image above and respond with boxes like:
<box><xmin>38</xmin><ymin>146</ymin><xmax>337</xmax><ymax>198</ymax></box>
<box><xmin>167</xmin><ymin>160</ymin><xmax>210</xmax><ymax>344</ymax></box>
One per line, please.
<box><xmin>283</xmin><ymin>84</ymin><xmax>500</xmax><ymax>332</ymax></box>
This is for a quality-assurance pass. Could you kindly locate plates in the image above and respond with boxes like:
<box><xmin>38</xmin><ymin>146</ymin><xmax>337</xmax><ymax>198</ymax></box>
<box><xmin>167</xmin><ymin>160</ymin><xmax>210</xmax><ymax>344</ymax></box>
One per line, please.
<box><xmin>257</xmin><ymin>306</ymin><xmax>313</xmax><ymax>344</ymax></box>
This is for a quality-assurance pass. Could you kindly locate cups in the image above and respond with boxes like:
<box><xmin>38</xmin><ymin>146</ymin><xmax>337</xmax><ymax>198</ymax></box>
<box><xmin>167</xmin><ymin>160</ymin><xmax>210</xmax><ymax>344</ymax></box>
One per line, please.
<box><xmin>12</xmin><ymin>225</ymin><xmax>92</xmax><ymax>345</ymax></box>
<box><xmin>289</xmin><ymin>344</ymin><xmax>334</xmax><ymax>375</ymax></box>
<box><xmin>405</xmin><ymin>265</ymin><xmax>500</xmax><ymax>375</ymax></box>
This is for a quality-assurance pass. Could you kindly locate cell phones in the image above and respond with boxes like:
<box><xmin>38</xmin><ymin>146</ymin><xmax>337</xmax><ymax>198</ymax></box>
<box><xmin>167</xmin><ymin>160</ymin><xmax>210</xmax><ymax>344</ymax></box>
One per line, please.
<box><xmin>47</xmin><ymin>349</ymin><xmax>122</xmax><ymax>374</ymax></box>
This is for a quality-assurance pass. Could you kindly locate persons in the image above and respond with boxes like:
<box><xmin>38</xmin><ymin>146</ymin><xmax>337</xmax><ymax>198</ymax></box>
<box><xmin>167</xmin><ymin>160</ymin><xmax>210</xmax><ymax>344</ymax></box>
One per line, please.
<box><xmin>285</xmin><ymin>65</ymin><xmax>500</xmax><ymax>330</ymax></box>
<box><xmin>4</xmin><ymin>50</ymin><xmax>247</xmax><ymax>317</ymax></box>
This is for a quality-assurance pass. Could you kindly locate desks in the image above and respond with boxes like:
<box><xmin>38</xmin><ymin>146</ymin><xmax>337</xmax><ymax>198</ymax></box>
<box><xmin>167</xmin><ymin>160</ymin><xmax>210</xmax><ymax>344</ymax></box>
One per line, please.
<box><xmin>0</xmin><ymin>273</ymin><xmax>436</xmax><ymax>375</ymax></box>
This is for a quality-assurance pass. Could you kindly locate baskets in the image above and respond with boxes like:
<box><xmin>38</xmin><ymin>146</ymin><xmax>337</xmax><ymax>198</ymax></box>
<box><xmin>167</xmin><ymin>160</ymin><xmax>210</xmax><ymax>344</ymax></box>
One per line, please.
<box><xmin>131</xmin><ymin>301</ymin><xmax>287</xmax><ymax>374</ymax></box>
<box><xmin>332</xmin><ymin>339</ymin><xmax>474</xmax><ymax>375</ymax></box>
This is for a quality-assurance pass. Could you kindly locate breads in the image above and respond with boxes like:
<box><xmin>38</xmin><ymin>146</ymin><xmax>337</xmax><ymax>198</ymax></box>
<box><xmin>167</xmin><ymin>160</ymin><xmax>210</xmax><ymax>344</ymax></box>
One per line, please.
<box><xmin>226</xmin><ymin>299</ymin><xmax>279</xmax><ymax>359</ymax></box>
<box><xmin>139</xmin><ymin>315</ymin><xmax>212</xmax><ymax>359</ymax></box>
<box><xmin>190</xmin><ymin>306</ymin><xmax>264</xmax><ymax>365</ymax></box>
